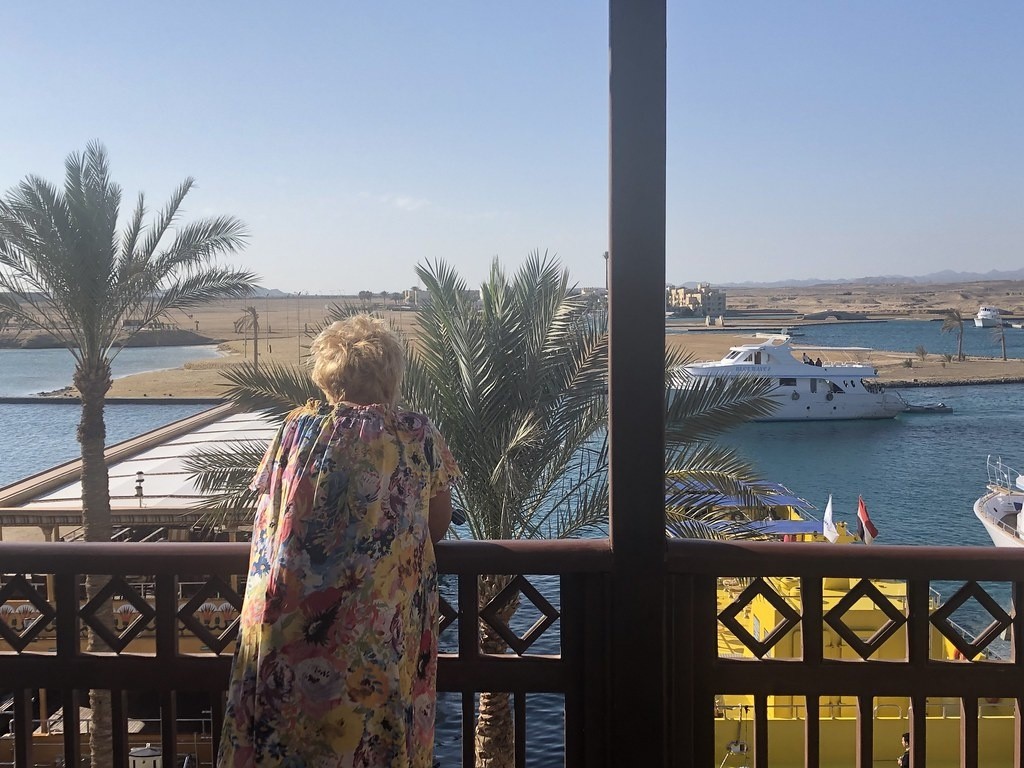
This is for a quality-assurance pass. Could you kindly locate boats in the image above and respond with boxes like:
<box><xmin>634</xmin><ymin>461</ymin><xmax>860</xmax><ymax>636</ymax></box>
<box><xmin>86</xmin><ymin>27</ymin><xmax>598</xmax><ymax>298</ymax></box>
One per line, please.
<box><xmin>973</xmin><ymin>452</ymin><xmax>1024</xmax><ymax>547</ymax></box>
<box><xmin>665</xmin><ymin>336</ymin><xmax>905</xmax><ymax>420</ymax></box>
<box><xmin>909</xmin><ymin>402</ymin><xmax>954</xmax><ymax>413</ymax></box>
<box><xmin>665</xmin><ymin>306</ymin><xmax>710</xmax><ymax>326</ymax></box>
<box><xmin>664</xmin><ymin>475</ymin><xmax>1015</xmax><ymax>768</ymax></box>
<box><xmin>974</xmin><ymin>306</ymin><xmax>1002</xmax><ymax>327</ymax></box>
<box><xmin>803</xmin><ymin>310</ymin><xmax>869</xmax><ymax>320</ymax></box>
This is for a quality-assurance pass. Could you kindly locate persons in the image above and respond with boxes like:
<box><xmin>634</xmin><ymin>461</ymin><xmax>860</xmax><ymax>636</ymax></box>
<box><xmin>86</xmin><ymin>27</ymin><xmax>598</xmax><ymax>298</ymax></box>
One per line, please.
<box><xmin>808</xmin><ymin>359</ymin><xmax>814</xmax><ymax>365</ymax></box>
<box><xmin>816</xmin><ymin>358</ymin><xmax>822</xmax><ymax>367</ymax></box>
<box><xmin>768</xmin><ymin>506</ymin><xmax>777</xmax><ymax>517</ymax></box>
<box><xmin>216</xmin><ymin>314</ymin><xmax>463</xmax><ymax>768</ymax></box>
<box><xmin>803</xmin><ymin>353</ymin><xmax>810</xmax><ymax>364</ymax></box>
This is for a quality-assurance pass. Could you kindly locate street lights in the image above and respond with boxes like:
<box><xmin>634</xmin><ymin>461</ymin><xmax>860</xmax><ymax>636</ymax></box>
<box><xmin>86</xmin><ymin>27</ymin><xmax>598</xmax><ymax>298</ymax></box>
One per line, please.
<box><xmin>603</xmin><ymin>251</ymin><xmax>609</xmax><ymax>290</ymax></box>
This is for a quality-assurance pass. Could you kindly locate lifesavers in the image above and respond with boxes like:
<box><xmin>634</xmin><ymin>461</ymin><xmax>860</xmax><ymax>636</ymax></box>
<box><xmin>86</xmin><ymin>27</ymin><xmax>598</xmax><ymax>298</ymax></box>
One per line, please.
<box><xmin>826</xmin><ymin>393</ymin><xmax>833</xmax><ymax>401</ymax></box>
<box><xmin>792</xmin><ymin>392</ymin><xmax>800</xmax><ymax>400</ymax></box>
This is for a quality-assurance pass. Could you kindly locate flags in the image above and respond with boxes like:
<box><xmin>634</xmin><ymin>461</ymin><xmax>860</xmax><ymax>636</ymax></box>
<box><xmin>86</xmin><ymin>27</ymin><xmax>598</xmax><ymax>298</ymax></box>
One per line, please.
<box><xmin>856</xmin><ymin>495</ymin><xmax>878</xmax><ymax>544</ymax></box>
<box><xmin>823</xmin><ymin>496</ymin><xmax>838</xmax><ymax>543</ymax></box>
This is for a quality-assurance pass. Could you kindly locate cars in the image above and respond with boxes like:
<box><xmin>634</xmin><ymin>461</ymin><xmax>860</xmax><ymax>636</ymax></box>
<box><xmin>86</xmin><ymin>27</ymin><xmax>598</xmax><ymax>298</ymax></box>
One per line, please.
<box><xmin>825</xmin><ymin>316</ymin><xmax>837</xmax><ymax>320</ymax></box>
